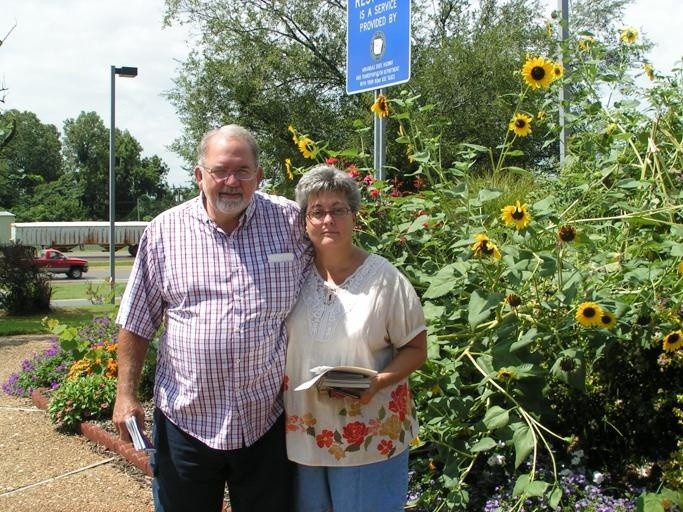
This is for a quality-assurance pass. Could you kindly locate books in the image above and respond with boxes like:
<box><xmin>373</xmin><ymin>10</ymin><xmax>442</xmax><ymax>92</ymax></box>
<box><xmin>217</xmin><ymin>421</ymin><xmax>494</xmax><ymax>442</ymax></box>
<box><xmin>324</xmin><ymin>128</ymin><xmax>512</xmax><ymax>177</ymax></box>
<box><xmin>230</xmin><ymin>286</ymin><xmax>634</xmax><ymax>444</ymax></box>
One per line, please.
<box><xmin>124</xmin><ymin>415</ymin><xmax>155</xmax><ymax>451</ymax></box>
<box><xmin>317</xmin><ymin>370</ymin><xmax>372</xmax><ymax>394</ymax></box>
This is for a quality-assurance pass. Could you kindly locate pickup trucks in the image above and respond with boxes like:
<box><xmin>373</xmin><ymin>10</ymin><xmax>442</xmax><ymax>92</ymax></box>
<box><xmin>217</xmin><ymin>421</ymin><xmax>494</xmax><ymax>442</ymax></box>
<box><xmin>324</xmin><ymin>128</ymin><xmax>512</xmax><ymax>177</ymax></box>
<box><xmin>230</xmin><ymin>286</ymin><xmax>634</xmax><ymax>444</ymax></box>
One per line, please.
<box><xmin>0</xmin><ymin>247</ymin><xmax>88</xmax><ymax>281</ymax></box>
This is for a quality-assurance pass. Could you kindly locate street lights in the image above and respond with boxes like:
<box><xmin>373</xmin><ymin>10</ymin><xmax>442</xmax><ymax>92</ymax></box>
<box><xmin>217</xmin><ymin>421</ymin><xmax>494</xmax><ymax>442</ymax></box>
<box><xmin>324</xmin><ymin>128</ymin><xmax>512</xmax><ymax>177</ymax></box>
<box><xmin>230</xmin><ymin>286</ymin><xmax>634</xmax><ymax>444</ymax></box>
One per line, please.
<box><xmin>109</xmin><ymin>64</ymin><xmax>137</xmax><ymax>303</ymax></box>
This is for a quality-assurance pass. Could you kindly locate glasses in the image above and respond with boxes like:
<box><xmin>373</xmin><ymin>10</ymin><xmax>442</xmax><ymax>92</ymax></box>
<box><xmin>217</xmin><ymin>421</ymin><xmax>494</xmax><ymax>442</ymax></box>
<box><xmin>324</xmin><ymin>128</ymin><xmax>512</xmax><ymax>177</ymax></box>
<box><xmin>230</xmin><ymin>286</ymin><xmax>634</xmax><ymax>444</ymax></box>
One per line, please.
<box><xmin>303</xmin><ymin>207</ymin><xmax>353</xmax><ymax>220</ymax></box>
<box><xmin>199</xmin><ymin>162</ymin><xmax>260</xmax><ymax>182</ymax></box>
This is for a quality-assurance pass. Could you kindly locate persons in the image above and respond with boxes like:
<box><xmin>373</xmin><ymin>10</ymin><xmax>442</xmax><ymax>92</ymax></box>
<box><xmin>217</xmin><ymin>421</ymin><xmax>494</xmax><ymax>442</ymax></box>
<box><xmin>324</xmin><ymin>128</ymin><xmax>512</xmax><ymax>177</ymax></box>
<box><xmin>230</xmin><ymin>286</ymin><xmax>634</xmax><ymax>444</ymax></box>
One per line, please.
<box><xmin>281</xmin><ymin>163</ymin><xmax>427</xmax><ymax>512</ymax></box>
<box><xmin>112</xmin><ymin>124</ymin><xmax>316</xmax><ymax>511</ymax></box>
